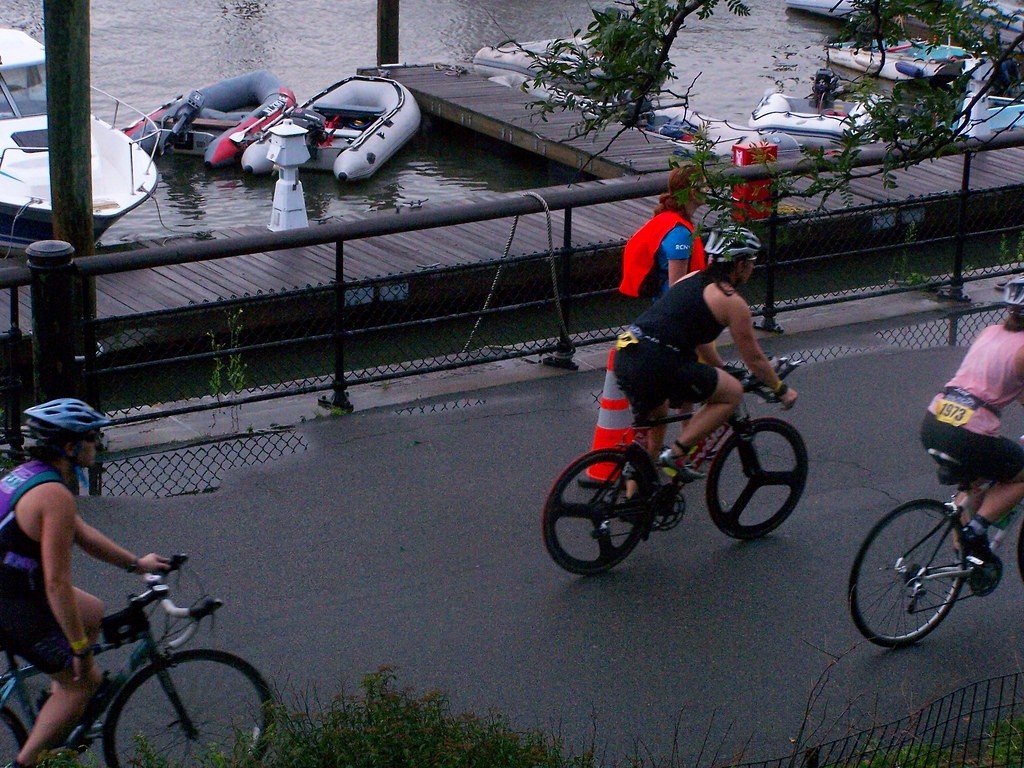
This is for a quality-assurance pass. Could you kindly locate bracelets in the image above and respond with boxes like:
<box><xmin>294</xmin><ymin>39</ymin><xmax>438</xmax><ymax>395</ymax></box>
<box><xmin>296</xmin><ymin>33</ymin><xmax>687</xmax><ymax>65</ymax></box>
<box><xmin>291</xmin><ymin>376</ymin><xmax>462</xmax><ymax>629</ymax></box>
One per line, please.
<box><xmin>773</xmin><ymin>380</ymin><xmax>782</xmax><ymax>395</ymax></box>
<box><xmin>72</xmin><ymin>648</ymin><xmax>91</xmax><ymax>659</ymax></box>
<box><xmin>70</xmin><ymin>638</ymin><xmax>88</xmax><ymax>651</ymax></box>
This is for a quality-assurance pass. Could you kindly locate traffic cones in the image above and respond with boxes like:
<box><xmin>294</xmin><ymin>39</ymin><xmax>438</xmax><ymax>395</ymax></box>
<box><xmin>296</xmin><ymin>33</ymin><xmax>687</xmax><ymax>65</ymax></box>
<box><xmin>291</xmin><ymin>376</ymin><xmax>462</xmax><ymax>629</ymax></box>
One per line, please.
<box><xmin>577</xmin><ymin>346</ymin><xmax>634</xmax><ymax>490</ymax></box>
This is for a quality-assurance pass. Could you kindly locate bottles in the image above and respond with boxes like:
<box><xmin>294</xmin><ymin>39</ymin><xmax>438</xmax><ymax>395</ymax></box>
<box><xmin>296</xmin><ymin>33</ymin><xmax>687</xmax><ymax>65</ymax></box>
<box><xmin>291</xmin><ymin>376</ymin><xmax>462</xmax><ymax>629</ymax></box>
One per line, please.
<box><xmin>663</xmin><ymin>446</ymin><xmax>698</xmax><ymax>477</ymax></box>
<box><xmin>992</xmin><ymin>506</ymin><xmax>1019</xmax><ymax>529</ymax></box>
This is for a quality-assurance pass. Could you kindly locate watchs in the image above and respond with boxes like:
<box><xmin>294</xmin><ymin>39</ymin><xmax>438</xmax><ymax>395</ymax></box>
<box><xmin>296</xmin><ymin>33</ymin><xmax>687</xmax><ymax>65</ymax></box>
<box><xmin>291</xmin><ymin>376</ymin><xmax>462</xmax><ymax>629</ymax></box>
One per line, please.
<box><xmin>777</xmin><ymin>382</ymin><xmax>789</xmax><ymax>398</ymax></box>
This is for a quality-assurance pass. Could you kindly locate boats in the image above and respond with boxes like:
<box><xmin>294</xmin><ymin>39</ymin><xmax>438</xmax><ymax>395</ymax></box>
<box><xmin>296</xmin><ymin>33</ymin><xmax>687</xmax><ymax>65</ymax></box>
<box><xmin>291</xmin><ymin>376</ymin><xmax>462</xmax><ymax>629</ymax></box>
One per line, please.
<box><xmin>616</xmin><ymin>107</ymin><xmax>801</xmax><ymax>162</ymax></box>
<box><xmin>241</xmin><ymin>74</ymin><xmax>422</xmax><ymax>182</ymax></box>
<box><xmin>747</xmin><ymin>67</ymin><xmax>886</xmax><ymax>151</ymax></box>
<box><xmin>786</xmin><ymin>1</ymin><xmax>1024</xmax><ymax>53</ymax></box>
<box><xmin>487</xmin><ymin>74</ymin><xmax>686</xmax><ymax>116</ymax></box>
<box><xmin>473</xmin><ymin>34</ymin><xmax>669</xmax><ymax>94</ymax></box>
<box><xmin>0</xmin><ymin>27</ymin><xmax>162</xmax><ymax>257</ymax></box>
<box><xmin>118</xmin><ymin>69</ymin><xmax>299</xmax><ymax>169</ymax></box>
<box><xmin>824</xmin><ymin>37</ymin><xmax>988</xmax><ymax>81</ymax></box>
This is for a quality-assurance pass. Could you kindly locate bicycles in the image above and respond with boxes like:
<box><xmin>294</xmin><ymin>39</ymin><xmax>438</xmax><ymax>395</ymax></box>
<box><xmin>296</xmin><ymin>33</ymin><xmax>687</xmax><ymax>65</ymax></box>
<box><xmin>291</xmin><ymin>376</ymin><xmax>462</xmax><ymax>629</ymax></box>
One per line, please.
<box><xmin>847</xmin><ymin>447</ymin><xmax>1024</xmax><ymax>649</ymax></box>
<box><xmin>0</xmin><ymin>553</ymin><xmax>275</xmax><ymax>768</ymax></box>
<box><xmin>541</xmin><ymin>356</ymin><xmax>810</xmax><ymax>575</ymax></box>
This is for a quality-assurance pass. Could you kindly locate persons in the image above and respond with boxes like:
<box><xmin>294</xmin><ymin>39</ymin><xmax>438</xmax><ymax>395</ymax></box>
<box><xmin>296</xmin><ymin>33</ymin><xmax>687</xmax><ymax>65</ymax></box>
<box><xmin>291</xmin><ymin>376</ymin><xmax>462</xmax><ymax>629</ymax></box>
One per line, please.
<box><xmin>920</xmin><ymin>275</ymin><xmax>1023</xmax><ymax>569</ymax></box>
<box><xmin>0</xmin><ymin>399</ymin><xmax>171</xmax><ymax>768</ymax></box>
<box><xmin>620</xmin><ymin>225</ymin><xmax>799</xmax><ymax>509</ymax></box>
<box><xmin>617</xmin><ymin>161</ymin><xmax>725</xmax><ymax>454</ymax></box>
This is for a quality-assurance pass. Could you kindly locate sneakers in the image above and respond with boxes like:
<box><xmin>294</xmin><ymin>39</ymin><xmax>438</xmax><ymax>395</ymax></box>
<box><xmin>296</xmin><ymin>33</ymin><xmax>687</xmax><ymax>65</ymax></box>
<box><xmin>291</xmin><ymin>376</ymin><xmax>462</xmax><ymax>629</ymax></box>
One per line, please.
<box><xmin>960</xmin><ymin>524</ymin><xmax>1002</xmax><ymax>575</ymax></box>
<box><xmin>657</xmin><ymin>447</ymin><xmax>707</xmax><ymax>486</ymax></box>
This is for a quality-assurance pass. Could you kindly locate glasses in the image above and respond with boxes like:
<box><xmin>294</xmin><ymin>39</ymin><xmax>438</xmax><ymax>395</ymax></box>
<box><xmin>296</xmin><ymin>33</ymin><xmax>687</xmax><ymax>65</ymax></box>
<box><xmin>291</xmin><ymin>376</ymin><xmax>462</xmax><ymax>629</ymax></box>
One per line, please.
<box><xmin>70</xmin><ymin>430</ymin><xmax>100</xmax><ymax>443</ymax></box>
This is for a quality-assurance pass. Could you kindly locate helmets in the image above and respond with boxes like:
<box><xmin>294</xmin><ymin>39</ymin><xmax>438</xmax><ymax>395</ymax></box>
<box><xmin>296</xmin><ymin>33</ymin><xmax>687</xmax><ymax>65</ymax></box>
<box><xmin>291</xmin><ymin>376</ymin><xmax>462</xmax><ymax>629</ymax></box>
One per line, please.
<box><xmin>1004</xmin><ymin>272</ymin><xmax>1024</xmax><ymax>315</ymax></box>
<box><xmin>18</xmin><ymin>396</ymin><xmax>114</xmax><ymax>457</ymax></box>
<box><xmin>705</xmin><ymin>224</ymin><xmax>761</xmax><ymax>264</ymax></box>
